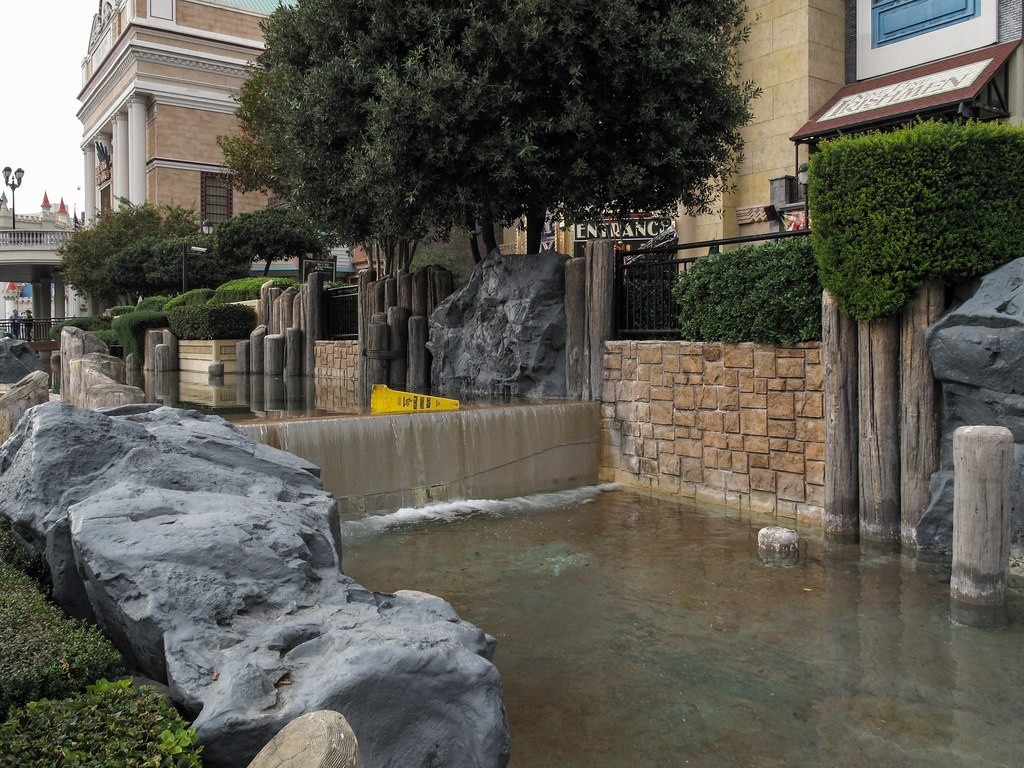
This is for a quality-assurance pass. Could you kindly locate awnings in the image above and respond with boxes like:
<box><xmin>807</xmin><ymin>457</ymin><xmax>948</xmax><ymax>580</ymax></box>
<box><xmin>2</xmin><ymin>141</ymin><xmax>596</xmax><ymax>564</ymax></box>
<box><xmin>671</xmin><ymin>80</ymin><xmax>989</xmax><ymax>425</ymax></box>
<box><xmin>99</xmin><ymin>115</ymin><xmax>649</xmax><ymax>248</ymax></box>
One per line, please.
<box><xmin>790</xmin><ymin>39</ymin><xmax>1021</xmax><ymax>141</ymax></box>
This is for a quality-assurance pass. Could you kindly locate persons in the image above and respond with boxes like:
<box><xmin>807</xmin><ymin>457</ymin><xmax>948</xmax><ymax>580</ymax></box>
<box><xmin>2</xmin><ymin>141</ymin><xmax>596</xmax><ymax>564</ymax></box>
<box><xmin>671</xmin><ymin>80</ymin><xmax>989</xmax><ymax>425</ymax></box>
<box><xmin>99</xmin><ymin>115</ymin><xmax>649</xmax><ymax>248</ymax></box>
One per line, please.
<box><xmin>9</xmin><ymin>309</ymin><xmax>21</xmax><ymax>339</ymax></box>
<box><xmin>24</xmin><ymin>310</ymin><xmax>33</xmax><ymax>341</ymax></box>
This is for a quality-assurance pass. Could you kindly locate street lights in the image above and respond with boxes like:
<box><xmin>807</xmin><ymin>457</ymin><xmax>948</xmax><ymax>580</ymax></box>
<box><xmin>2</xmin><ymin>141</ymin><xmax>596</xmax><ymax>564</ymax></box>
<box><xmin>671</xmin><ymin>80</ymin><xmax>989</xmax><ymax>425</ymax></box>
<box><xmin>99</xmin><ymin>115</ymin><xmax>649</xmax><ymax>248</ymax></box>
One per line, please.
<box><xmin>2</xmin><ymin>166</ymin><xmax>25</xmax><ymax>230</ymax></box>
<box><xmin>181</xmin><ymin>220</ymin><xmax>216</xmax><ymax>291</ymax></box>
<box><xmin>799</xmin><ymin>160</ymin><xmax>812</xmax><ymax>230</ymax></box>
